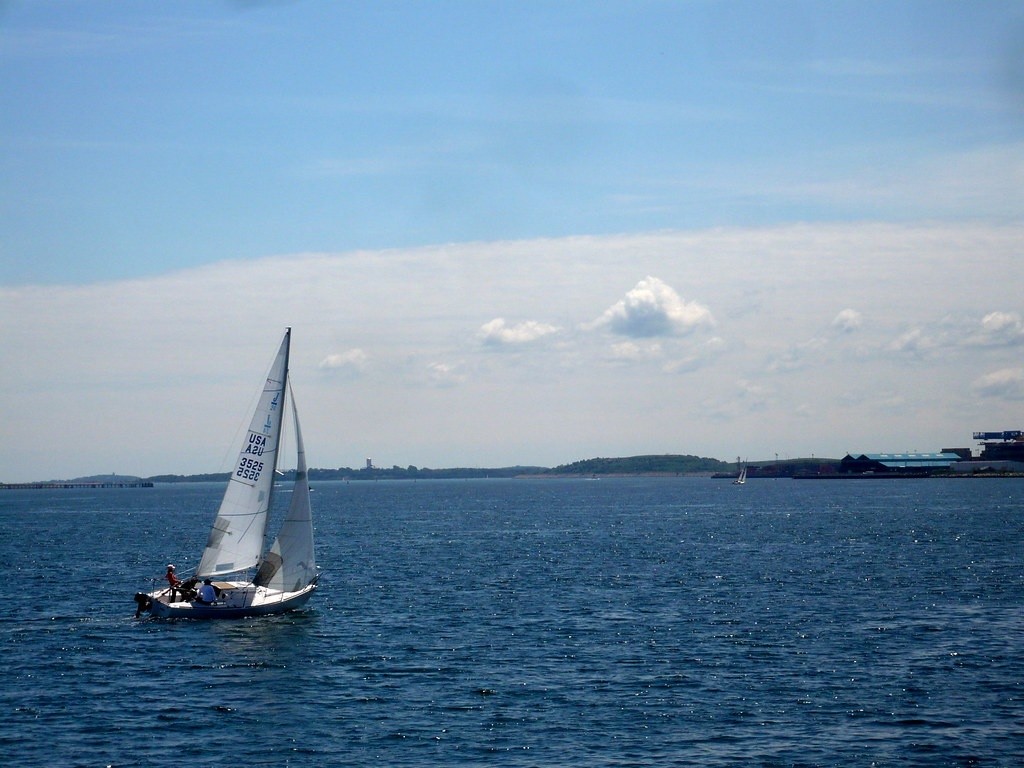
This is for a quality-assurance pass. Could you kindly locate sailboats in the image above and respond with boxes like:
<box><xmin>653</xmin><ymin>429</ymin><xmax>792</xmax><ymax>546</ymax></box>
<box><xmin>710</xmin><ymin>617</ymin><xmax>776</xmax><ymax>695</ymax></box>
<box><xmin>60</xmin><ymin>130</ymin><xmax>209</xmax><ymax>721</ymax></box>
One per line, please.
<box><xmin>732</xmin><ymin>462</ymin><xmax>747</xmax><ymax>484</ymax></box>
<box><xmin>131</xmin><ymin>327</ymin><xmax>325</xmax><ymax>619</ymax></box>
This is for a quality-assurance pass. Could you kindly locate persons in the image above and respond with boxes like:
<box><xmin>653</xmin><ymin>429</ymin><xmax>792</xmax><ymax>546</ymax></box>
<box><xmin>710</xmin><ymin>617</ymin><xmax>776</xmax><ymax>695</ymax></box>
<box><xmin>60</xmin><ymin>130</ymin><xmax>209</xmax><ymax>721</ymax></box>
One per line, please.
<box><xmin>165</xmin><ymin>565</ymin><xmax>198</xmax><ymax>602</ymax></box>
<box><xmin>195</xmin><ymin>580</ymin><xmax>218</xmax><ymax>605</ymax></box>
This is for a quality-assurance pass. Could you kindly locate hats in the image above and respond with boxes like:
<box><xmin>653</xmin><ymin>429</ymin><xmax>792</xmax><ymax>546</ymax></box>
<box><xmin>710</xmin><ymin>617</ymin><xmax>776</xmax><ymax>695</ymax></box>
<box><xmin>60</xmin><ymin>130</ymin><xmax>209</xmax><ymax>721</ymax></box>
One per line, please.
<box><xmin>204</xmin><ymin>579</ymin><xmax>212</xmax><ymax>584</ymax></box>
<box><xmin>167</xmin><ymin>565</ymin><xmax>175</xmax><ymax>570</ymax></box>
